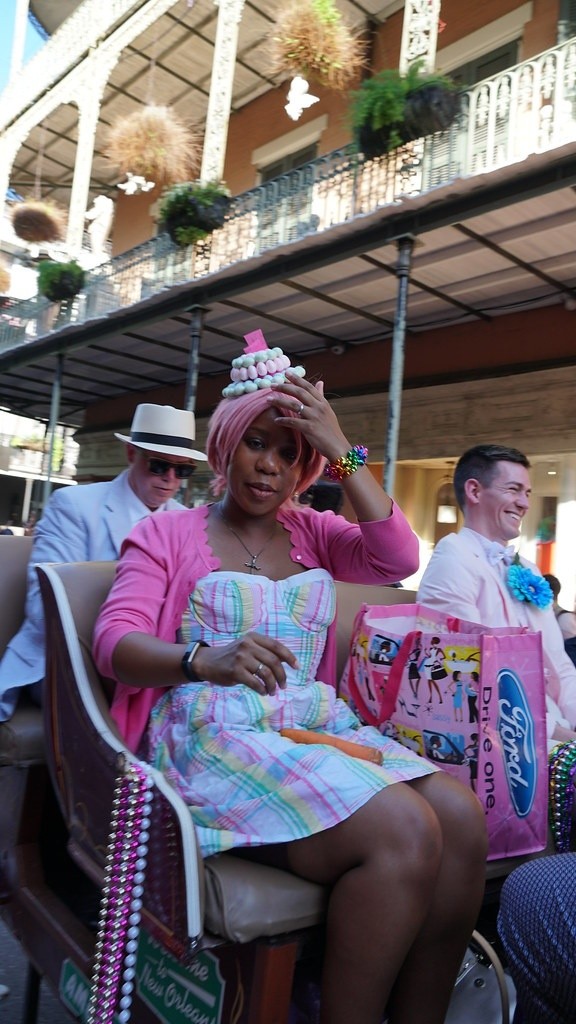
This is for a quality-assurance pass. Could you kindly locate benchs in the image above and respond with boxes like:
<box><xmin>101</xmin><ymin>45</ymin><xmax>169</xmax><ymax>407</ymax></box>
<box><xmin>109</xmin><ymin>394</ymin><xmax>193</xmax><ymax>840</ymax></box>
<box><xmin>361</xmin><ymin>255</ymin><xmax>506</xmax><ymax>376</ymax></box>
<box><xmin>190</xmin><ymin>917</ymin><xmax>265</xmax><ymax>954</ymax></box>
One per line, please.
<box><xmin>0</xmin><ymin>535</ymin><xmax>556</xmax><ymax>1023</ymax></box>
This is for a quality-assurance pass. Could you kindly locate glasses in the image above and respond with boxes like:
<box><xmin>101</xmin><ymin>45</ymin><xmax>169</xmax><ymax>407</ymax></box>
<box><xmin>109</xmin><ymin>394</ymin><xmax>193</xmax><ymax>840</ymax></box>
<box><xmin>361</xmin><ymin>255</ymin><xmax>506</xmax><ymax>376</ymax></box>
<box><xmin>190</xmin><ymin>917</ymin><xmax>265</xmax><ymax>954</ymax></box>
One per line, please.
<box><xmin>136</xmin><ymin>447</ymin><xmax>198</xmax><ymax>479</ymax></box>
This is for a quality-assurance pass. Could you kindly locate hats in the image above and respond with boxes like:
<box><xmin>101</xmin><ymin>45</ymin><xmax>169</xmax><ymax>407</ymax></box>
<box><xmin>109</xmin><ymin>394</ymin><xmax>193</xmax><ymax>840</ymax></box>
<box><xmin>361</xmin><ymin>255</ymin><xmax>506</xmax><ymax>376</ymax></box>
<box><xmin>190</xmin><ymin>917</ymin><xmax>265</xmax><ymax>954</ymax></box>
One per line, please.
<box><xmin>114</xmin><ymin>403</ymin><xmax>210</xmax><ymax>462</ymax></box>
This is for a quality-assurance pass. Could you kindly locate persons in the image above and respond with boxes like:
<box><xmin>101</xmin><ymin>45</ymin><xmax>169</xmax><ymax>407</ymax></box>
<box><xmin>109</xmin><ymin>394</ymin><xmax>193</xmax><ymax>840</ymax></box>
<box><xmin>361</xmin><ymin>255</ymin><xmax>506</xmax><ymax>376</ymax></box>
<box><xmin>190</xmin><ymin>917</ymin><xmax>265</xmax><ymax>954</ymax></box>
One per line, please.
<box><xmin>543</xmin><ymin>574</ymin><xmax>576</xmax><ymax>668</ymax></box>
<box><xmin>0</xmin><ymin>402</ymin><xmax>208</xmax><ymax>721</ymax></box>
<box><xmin>6</xmin><ymin>511</ymin><xmax>25</xmax><ymax>526</ymax></box>
<box><xmin>89</xmin><ymin>329</ymin><xmax>486</xmax><ymax>1024</ymax></box>
<box><xmin>496</xmin><ymin>853</ymin><xmax>576</xmax><ymax>1024</ymax></box>
<box><xmin>418</xmin><ymin>444</ymin><xmax>576</xmax><ymax>755</ymax></box>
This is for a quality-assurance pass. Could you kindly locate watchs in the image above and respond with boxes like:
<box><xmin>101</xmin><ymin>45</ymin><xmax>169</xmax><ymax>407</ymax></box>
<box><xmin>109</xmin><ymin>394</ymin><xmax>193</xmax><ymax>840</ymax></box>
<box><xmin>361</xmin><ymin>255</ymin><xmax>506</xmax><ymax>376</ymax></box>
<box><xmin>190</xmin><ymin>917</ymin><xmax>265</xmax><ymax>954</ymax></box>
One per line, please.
<box><xmin>181</xmin><ymin>640</ymin><xmax>209</xmax><ymax>683</ymax></box>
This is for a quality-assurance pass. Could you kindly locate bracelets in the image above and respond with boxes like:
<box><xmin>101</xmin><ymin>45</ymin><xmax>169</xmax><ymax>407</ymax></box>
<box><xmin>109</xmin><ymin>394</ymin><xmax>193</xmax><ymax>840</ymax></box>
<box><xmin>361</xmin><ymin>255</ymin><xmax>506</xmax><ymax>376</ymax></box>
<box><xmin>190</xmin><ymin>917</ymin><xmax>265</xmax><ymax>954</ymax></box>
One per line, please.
<box><xmin>324</xmin><ymin>445</ymin><xmax>368</xmax><ymax>481</ymax></box>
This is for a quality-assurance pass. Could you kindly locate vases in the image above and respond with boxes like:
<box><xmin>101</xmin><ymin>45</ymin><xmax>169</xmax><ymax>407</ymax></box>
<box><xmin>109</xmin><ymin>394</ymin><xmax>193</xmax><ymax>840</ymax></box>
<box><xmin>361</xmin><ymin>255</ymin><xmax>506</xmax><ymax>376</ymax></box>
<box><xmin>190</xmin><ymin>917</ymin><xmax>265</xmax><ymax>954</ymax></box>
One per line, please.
<box><xmin>170</xmin><ymin>195</ymin><xmax>227</xmax><ymax>245</ymax></box>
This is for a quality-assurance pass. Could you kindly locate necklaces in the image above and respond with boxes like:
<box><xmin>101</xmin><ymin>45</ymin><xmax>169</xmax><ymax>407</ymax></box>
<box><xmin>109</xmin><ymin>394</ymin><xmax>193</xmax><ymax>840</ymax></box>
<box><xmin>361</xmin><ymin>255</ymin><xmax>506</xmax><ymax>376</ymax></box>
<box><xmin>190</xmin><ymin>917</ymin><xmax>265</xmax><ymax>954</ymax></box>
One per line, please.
<box><xmin>547</xmin><ymin>738</ymin><xmax>576</xmax><ymax>853</ymax></box>
<box><xmin>218</xmin><ymin>502</ymin><xmax>274</xmax><ymax>574</ymax></box>
<box><xmin>553</xmin><ymin>605</ymin><xmax>560</xmax><ymax>611</ymax></box>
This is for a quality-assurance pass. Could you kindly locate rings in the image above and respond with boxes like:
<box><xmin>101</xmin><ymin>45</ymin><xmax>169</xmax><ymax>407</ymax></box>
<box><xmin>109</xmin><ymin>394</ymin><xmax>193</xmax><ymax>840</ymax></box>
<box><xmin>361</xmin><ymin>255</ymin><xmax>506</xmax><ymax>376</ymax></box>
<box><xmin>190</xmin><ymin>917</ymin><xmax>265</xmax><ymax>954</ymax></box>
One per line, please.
<box><xmin>256</xmin><ymin>663</ymin><xmax>264</xmax><ymax>675</ymax></box>
<box><xmin>298</xmin><ymin>404</ymin><xmax>303</xmax><ymax>414</ymax></box>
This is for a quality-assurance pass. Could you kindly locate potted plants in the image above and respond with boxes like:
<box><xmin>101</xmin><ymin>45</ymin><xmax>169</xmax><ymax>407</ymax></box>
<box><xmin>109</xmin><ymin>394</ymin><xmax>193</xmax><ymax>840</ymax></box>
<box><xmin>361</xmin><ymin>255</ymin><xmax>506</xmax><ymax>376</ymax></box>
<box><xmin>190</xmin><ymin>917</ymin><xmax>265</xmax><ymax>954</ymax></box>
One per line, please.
<box><xmin>38</xmin><ymin>260</ymin><xmax>84</xmax><ymax>300</ymax></box>
<box><xmin>348</xmin><ymin>58</ymin><xmax>473</xmax><ymax>161</ymax></box>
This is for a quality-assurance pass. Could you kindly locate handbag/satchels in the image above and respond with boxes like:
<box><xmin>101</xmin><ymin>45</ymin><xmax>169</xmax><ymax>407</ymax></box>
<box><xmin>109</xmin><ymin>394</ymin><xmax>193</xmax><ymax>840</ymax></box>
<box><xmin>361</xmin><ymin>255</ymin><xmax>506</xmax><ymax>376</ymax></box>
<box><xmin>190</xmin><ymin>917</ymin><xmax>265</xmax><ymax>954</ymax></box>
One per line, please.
<box><xmin>339</xmin><ymin>602</ymin><xmax>546</xmax><ymax>862</ymax></box>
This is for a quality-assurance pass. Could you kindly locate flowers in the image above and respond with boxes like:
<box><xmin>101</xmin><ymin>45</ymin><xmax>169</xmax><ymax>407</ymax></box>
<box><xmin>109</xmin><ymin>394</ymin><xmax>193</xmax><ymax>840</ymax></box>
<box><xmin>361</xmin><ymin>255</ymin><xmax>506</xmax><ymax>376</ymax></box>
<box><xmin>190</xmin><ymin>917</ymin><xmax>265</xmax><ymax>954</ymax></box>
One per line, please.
<box><xmin>509</xmin><ymin>563</ymin><xmax>553</xmax><ymax>609</ymax></box>
<box><xmin>154</xmin><ymin>178</ymin><xmax>230</xmax><ymax>246</ymax></box>
